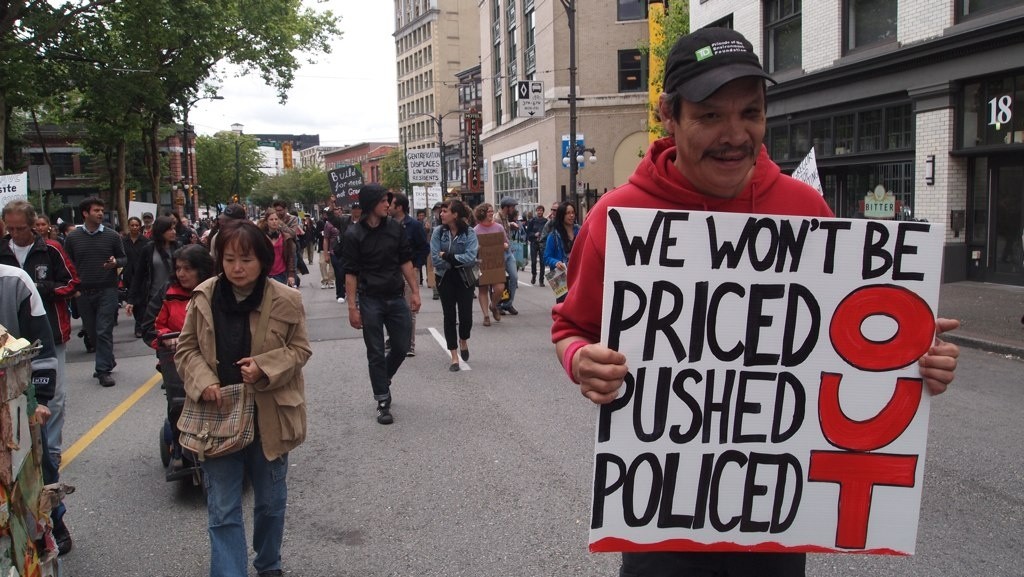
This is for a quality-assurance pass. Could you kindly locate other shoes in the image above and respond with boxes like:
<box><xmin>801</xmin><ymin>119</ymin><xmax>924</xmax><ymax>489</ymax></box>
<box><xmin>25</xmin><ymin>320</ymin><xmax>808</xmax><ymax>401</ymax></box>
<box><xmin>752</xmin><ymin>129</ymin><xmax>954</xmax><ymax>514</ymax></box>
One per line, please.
<box><xmin>502</xmin><ymin>305</ymin><xmax>518</xmax><ymax>315</ymax></box>
<box><xmin>328</xmin><ymin>279</ymin><xmax>336</xmax><ymax>289</ymax></box>
<box><xmin>449</xmin><ymin>363</ymin><xmax>459</xmax><ymax>371</ymax></box>
<box><xmin>337</xmin><ymin>296</ymin><xmax>344</xmax><ymax>303</ymax></box>
<box><xmin>531</xmin><ymin>276</ymin><xmax>536</xmax><ymax>284</ymax></box>
<box><xmin>489</xmin><ymin>303</ymin><xmax>500</xmax><ymax>321</ymax></box>
<box><xmin>500</xmin><ymin>310</ymin><xmax>506</xmax><ymax>315</ymax></box>
<box><xmin>483</xmin><ymin>316</ymin><xmax>490</xmax><ymax>326</ymax></box>
<box><xmin>320</xmin><ymin>281</ymin><xmax>329</xmax><ymax>289</ymax></box>
<box><xmin>98</xmin><ymin>373</ymin><xmax>115</xmax><ymax>386</ymax></box>
<box><xmin>540</xmin><ymin>283</ymin><xmax>546</xmax><ymax>287</ymax></box>
<box><xmin>459</xmin><ymin>339</ymin><xmax>469</xmax><ymax>361</ymax></box>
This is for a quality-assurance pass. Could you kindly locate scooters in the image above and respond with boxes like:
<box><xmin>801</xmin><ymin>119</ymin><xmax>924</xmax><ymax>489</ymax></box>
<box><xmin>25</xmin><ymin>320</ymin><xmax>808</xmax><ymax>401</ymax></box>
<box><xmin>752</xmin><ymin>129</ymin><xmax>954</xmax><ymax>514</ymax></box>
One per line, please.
<box><xmin>159</xmin><ymin>330</ymin><xmax>252</xmax><ymax>498</ymax></box>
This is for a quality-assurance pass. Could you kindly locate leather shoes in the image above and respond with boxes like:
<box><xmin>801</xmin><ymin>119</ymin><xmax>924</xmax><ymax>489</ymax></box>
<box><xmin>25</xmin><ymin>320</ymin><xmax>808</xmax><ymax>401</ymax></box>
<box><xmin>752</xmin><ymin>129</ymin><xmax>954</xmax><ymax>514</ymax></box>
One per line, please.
<box><xmin>52</xmin><ymin>519</ymin><xmax>72</xmax><ymax>555</ymax></box>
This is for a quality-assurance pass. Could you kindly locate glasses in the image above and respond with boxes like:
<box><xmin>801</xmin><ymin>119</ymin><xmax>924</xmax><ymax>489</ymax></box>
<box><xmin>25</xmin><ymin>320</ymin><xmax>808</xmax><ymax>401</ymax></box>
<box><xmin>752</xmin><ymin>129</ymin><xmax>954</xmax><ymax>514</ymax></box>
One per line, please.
<box><xmin>551</xmin><ymin>208</ymin><xmax>558</xmax><ymax>211</ymax></box>
<box><xmin>566</xmin><ymin>211</ymin><xmax>576</xmax><ymax>214</ymax></box>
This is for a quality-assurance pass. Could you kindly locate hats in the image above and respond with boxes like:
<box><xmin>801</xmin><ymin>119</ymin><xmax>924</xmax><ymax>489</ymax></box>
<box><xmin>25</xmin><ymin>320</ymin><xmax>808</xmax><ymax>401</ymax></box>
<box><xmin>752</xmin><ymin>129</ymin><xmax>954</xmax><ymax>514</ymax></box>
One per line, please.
<box><xmin>431</xmin><ymin>201</ymin><xmax>443</xmax><ymax>210</ymax></box>
<box><xmin>501</xmin><ymin>196</ymin><xmax>518</xmax><ymax>208</ymax></box>
<box><xmin>663</xmin><ymin>26</ymin><xmax>779</xmax><ymax>104</ymax></box>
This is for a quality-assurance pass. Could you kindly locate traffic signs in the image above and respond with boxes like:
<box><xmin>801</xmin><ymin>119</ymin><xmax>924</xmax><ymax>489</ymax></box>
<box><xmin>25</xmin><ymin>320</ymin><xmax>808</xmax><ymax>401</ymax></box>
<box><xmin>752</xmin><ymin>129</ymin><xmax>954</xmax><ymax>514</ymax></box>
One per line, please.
<box><xmin>517</xmin><ymin>80</ymin><xmax>546</xmax><ymax>119</ymax></box>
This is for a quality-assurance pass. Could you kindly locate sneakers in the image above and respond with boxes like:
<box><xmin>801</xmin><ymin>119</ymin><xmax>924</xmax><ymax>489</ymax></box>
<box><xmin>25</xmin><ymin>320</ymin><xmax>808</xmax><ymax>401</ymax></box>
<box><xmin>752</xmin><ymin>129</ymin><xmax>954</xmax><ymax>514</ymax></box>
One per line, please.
<box><xmin>376</xmin><ymin>402</ymin><xmax>393</xmax><ymax>424</ymax></box>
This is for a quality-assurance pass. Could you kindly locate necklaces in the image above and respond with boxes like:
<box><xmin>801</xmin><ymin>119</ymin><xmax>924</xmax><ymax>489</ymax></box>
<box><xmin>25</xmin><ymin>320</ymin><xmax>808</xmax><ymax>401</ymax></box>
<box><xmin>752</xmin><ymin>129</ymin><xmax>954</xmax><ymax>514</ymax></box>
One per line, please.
<box><xmin>13</xmin><ymin>234</ymin><xmax>34</xmax><ymax>266</ymax></box>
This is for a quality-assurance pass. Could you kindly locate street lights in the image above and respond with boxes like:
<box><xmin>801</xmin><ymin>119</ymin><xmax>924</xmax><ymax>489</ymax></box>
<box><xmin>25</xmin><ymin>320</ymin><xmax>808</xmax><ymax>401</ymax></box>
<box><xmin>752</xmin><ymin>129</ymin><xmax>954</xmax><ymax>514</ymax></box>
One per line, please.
<box><xmin>234</xmin><ymin>137</ymin><xmax>260</xmax><ymax>205</ymax></box>
<box><xmin>408</xmin><ymin>109</ymin><xmax>469</xmax><ymax>201</ymax></box>
<box><xmin>173</xmin><ymin>96</ymin><xmax>225</xmax><ymax>219</ymax></box>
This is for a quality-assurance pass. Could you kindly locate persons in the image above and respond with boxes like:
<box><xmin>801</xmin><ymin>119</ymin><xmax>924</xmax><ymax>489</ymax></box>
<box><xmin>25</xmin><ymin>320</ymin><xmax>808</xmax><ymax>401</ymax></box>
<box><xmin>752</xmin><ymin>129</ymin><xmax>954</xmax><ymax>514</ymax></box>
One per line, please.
<box><xmin>151</xmin><ymin>243</ymin><xmax>219</xmax><ymax>482</ymax></box>
<box><xmin>0</xmin><ymin>195</ymin><xmax>585</xmax><ymax>372</ymax></box>
<box><xmin>343</xmin><ymin>183</ymin><xmax>422</xmax><ymax>425</ymax></box>
<box><xmin>0</xmin><ymin>200</ymin><xmax>81</xmax><ymax>483</ymax></box>
<box><xmin>552</xmin><ymin>27</ymin><xmax>959</xmax><ymax>577</ymax></box>
<box><xmin>64</xmin><ymin>197</ymin><xmax>128</xmax><ymax>388</ymax></box>
<box><xmin>0</xmin><ymin>264</ymin><xmax>72</xmax><ymax>556</ymax></box>
<box><xmin>173</xmin><ymin>220</ymin><xmax>313</xmax><ymax>577</ymax></box>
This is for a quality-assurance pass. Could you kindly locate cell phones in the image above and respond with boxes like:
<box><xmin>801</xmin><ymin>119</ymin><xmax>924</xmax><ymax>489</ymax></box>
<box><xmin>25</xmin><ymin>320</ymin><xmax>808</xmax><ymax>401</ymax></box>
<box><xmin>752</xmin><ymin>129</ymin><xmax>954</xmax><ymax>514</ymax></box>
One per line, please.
<box><xmin>105</xmin><ymin>259</ymin><xmax>112</xmax><ymax>264</ymax></box>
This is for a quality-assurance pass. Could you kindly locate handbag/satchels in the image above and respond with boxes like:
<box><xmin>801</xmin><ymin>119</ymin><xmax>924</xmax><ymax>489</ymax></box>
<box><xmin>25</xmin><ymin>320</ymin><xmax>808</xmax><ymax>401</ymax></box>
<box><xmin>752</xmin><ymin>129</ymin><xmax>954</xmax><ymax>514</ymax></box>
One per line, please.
<box><xmin>459</xmin><ymin>261</ymin><xmax>484</xmax><ymax>288</ymax></box>
<box><xmin>176</xmin><ymin>382</ymin><xmax>255</xmax><ymax>462</ymax></box>
<box><xmin>489</xmin><ymin>276</ymin><xmax>518</xmax><ymax>304</ymax></box>
